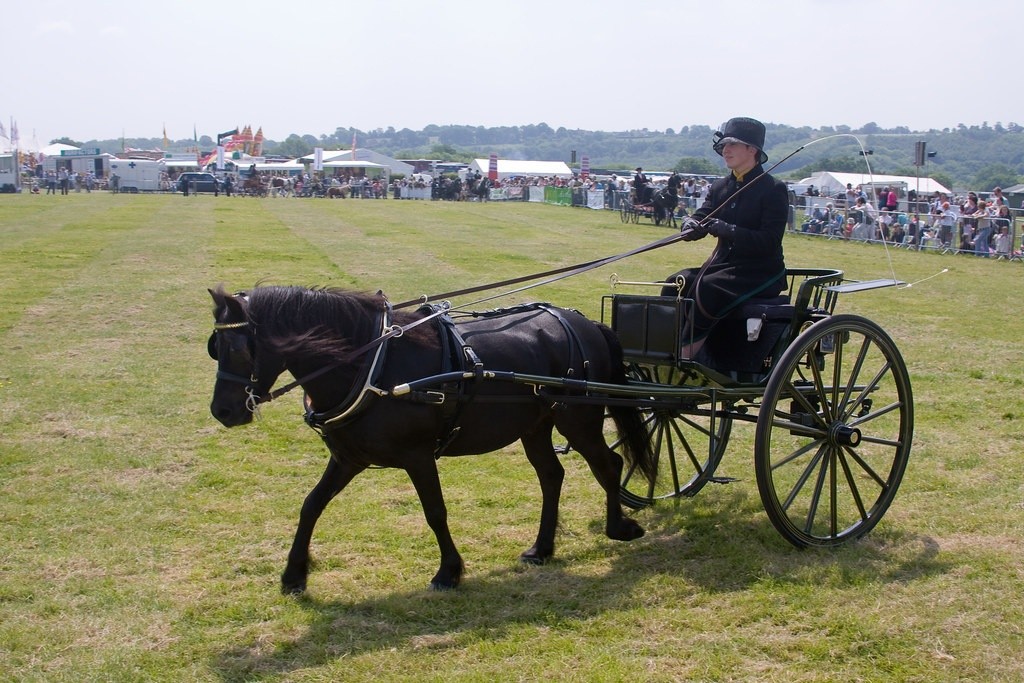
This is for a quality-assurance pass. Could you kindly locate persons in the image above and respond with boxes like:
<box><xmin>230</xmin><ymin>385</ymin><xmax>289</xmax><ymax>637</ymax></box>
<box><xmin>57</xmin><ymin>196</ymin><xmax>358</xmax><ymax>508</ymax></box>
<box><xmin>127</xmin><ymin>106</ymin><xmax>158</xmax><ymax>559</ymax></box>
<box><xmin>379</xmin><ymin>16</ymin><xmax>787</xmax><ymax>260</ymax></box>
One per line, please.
<box><xmin>660</xmin><ymin>117</ymin><xmax>788</xmax><ymax>377</ymax></box>
<box><xmin>19</xmin><ymin>163</ymin><xmax>1014</xmax><ymax>264</ymax></box>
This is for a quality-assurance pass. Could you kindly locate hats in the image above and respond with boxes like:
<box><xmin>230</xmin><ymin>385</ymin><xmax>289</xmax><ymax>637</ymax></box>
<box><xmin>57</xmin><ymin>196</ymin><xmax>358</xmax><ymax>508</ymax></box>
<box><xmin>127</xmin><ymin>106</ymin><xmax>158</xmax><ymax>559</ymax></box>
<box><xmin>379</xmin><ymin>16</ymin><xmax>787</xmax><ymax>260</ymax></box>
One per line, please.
<box><xmin>475</xmin><ymin>169</ymin><xmax>480</xmax><ymax>172</ymax></box>
<box><xmin>635</xmin><ymin>167</ymin><xmax>642</xmax><ymax>171</ymax></box>
<box><xmin>468</xmin><ymin>167</ymin><xmax>472</xmax><ymax>170</ymax></box>
<box><xmin>813</xmin><ymin>204</ymin><xmax>819</xmax><ymax>207</ymax></box>
<box><xmin>846</xmin><ymin>217</ymin><xmax>855</xmax><ymax>224</ymax></box>
<box><xmin>610</xmin><ymin>173</ymin><xmax>617</xmax><ymax>178</ymax></box>
<box><xmin>826</xmin><ymin>203</ymin><xmax>833</xmax><ymax>207</ymax></box>
<box><xmin>942</xmin><ymin>202</ymin><xmax>950</xmax><ymax>208</ymax></box>
<box><xmin>712</xmin><ymin>117</ymin><xmax>768</xmax><ymax>165</ymax></box>
<box><xmin>484</xmin><ymin>177</ymin><xmax>489</xmax><ymax>180</ymax></box>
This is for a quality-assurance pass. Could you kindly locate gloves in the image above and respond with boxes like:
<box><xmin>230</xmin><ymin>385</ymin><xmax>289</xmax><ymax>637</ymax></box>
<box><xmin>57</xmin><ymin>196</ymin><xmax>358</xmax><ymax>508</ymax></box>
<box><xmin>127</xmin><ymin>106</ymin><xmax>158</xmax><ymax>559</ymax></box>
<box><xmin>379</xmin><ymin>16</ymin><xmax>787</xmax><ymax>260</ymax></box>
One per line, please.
<box><xmin>704</xmin><ymin>217</ymin><xmax>735</xmax><ymax>238</ymax></box>
<box><xmin>680</xmin><ymin>218</ymin><xmax>707</xmax><ymax>242</ymax></box>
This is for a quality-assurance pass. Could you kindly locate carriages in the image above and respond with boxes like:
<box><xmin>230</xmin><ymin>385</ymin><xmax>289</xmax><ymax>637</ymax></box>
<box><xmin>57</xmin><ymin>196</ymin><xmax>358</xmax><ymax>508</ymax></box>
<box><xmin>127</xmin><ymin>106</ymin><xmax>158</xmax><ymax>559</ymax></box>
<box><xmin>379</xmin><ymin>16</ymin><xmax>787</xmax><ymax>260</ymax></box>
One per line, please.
<box><xmin>206</xmin><ymin>218</ymin><xmax>915</xmax><ymax>597</ymax></box>
<box><xmin>438</xmin><ymin>178</ymin><xmax>490</xmax><ymax>202</ymax></box>
<box><xmin>238</xmin><ymin>170</ymin><xmax>299</xmax><ymax>198</ymax></box>
<box><xmin>620</xmin><ymin>171</ymin><xmax>681</xmax><ymax>228</ymax></box>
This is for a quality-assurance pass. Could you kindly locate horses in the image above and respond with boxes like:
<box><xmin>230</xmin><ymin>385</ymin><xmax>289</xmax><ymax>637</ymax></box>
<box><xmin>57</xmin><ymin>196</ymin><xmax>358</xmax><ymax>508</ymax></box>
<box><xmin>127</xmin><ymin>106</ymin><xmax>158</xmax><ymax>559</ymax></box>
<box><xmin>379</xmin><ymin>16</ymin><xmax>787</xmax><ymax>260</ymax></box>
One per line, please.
<box><xmin>651</xmin><ymin>170</ymin><xmax>683</xmax><ymax>229</ymax></box>
<box><xmin>270</xmin><ymin>176</ymin><xmax>299</xmax><ymax>200</ymax></box>
<box><xmin>204</xmin><ymin>280</ymin><xmax>670</xmax><ymax>597</ymax></box>
<box><xmin>327</xmin><ymin>184</ymin><xmax>352</xmax><ymax>199</ymax></box>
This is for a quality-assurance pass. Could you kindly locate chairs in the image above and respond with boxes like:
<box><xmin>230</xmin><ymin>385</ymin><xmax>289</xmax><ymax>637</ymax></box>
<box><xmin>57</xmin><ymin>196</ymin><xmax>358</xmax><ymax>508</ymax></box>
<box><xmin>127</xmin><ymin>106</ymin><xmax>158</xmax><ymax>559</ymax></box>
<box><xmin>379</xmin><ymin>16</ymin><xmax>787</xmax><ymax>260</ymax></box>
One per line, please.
<box><xmin>827</xmin><ymin>217</ymin><xmax>941</xmax><ymax>254</ymax></box>
<box><xmin>627</xmin><ymin>179</ymin><xmax>651</xmax><ymax>196</ymax></box>
<box><xmin>707</xmin><ymin>268</ymin><xmax>843</xmax><ymax>368</ymax></box>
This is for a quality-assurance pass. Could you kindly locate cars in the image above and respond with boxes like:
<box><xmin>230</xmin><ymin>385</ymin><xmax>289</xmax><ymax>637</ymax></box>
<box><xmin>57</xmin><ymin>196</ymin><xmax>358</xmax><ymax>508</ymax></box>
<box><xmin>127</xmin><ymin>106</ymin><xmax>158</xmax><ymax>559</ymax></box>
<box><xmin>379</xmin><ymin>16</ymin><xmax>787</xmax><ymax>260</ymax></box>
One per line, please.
<box><xmin>176</xmin><ymin>172</ymin><xmax>234</xmax><ymax>192</ymax></box>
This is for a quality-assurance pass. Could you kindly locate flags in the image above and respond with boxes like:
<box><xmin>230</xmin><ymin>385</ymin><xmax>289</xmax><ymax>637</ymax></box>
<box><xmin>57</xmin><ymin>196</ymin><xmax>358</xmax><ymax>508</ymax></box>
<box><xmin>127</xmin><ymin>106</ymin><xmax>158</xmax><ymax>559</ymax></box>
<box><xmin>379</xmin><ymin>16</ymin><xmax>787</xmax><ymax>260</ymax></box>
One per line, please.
<box><xmin>0</xmin><ymin>117</ymin><xmax>356</xmax><ymax>161</ymax></box>
